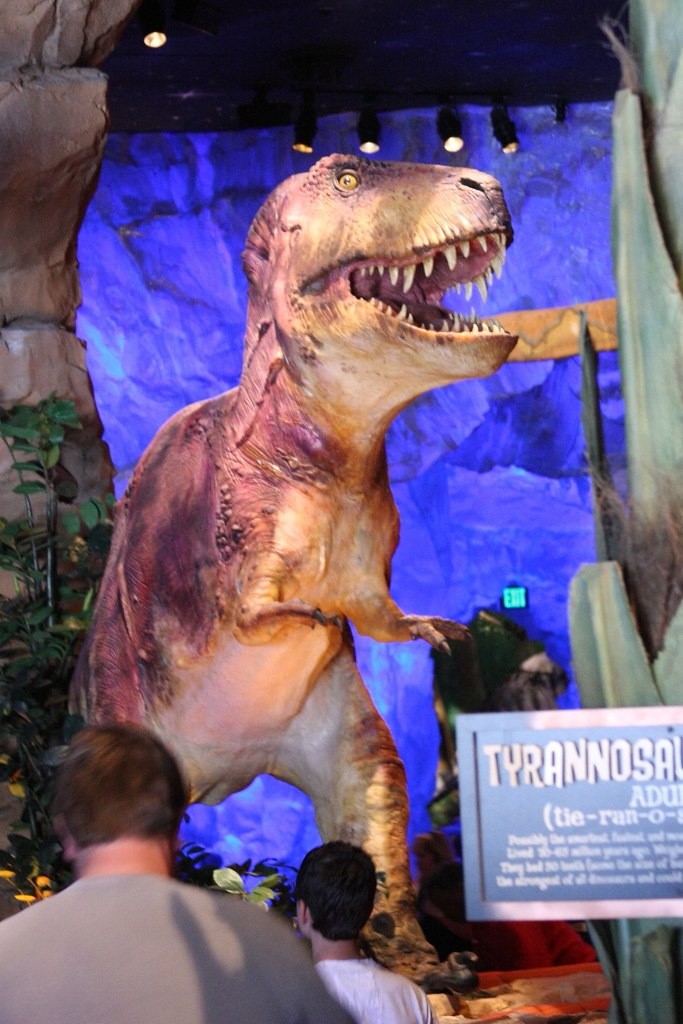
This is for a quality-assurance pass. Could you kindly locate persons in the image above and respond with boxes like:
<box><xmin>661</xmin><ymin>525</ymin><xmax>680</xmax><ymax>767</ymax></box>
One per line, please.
<box><xmin>292</xmin><ymin>840</ymin><xmax>431</xmax><ymax>1024</ymax></box>
<box><xmin>1</xmin><ymin>718</ymin><xmax>361</xmax><ymax>1024</ymax></box>
